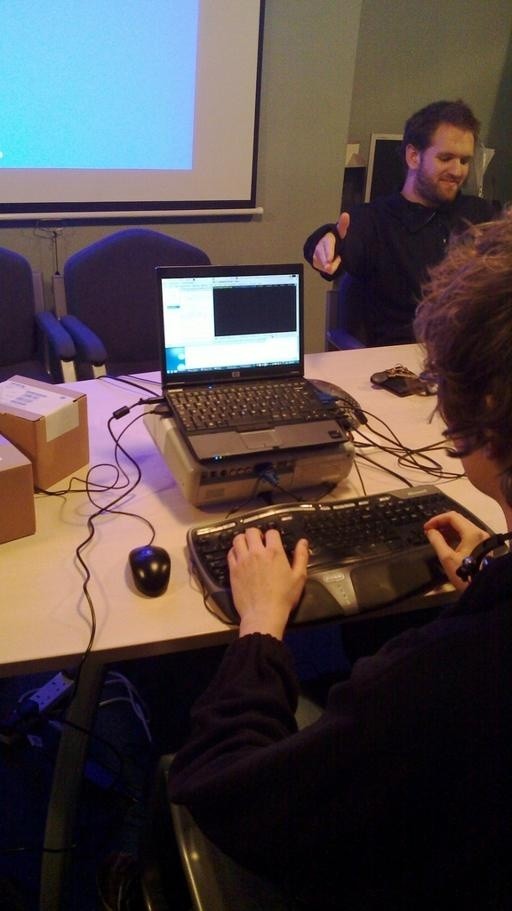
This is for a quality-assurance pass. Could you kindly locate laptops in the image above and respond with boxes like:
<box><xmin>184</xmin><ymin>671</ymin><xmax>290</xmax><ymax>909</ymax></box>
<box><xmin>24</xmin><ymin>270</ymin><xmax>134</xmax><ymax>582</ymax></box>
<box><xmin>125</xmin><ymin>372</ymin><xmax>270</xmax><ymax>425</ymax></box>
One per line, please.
<box><xmin>154</xmin><ymin>263</ymin><xmax>349</xmax><ymax>463</ymax></box>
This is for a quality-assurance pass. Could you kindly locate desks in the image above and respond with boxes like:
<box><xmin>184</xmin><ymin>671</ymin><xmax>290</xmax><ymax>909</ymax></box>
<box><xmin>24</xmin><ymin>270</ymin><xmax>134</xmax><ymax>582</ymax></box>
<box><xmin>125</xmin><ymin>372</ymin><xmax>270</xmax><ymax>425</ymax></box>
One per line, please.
<box><xmin>1</xmin><ymin>343</ymin><xmax>512</xmax><ymax>910</ymax></box>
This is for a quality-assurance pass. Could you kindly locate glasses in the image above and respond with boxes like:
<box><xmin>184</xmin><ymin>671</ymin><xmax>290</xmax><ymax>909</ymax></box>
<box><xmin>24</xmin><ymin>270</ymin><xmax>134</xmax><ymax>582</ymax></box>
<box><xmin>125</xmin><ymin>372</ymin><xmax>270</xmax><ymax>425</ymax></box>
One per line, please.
<box><xmin>418</xmin><ymin>368</ymin><xmax>440</xmax><ymax>398</ymax></box>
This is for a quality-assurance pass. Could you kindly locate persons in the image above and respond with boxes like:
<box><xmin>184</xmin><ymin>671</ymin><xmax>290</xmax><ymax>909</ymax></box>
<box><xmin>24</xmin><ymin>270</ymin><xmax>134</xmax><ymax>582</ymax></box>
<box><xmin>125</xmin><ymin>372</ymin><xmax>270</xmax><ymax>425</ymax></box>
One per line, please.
<box><xmin>166</xmin><ymin>203</ymin><xmax>511</xmax><ymax>909</ymax></box>
<box><xmin>302</xmin><ymin>98</ymin><xmax>504</xmax><ymax>349</ymax></box>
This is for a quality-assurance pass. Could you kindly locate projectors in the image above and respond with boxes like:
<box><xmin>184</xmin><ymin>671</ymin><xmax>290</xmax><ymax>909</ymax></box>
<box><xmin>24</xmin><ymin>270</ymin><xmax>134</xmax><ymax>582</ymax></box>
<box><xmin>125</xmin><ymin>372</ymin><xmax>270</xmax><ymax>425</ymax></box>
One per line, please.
<box><xmin>142</xmin><ymin>403</ymin><xmax>352</xmax><ymax>507</ymax></box>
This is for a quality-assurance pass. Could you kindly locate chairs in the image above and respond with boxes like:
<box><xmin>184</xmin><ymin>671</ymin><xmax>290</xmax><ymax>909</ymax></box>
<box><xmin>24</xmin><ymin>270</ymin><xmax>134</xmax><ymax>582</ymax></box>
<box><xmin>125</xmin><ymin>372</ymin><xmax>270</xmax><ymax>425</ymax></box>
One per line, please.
<box><xmin>324</xmin><ymin>290</ymin><xmax>367</xmax><ymax>351</ymax></box>
<box><xmin>117</xmin><ymin>697</ymin><xmax>322</xmax><ymax>909</ymax></box>
<box><xmin>1</xmin><ymin>244</ymin><xmax>78</xmax><ymax>388</ymax></box>
<box><xmin>53</xmin><ymin>226</ymin><xmax>213</xmax><ymax>380</ymax></box>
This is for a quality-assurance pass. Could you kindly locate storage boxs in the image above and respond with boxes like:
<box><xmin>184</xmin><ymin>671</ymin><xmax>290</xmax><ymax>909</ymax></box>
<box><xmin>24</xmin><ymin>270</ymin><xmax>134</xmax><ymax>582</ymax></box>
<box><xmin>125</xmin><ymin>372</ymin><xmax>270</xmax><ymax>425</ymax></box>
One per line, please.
<box><xmin>0</xmin><ymin>374</ymin><xmax>91</xmax><ymax>491</ymax></box>
<box><xmin>0</xmin><ymin>432</ymin><xmax>36</xmax><ymax>544</ymax></box>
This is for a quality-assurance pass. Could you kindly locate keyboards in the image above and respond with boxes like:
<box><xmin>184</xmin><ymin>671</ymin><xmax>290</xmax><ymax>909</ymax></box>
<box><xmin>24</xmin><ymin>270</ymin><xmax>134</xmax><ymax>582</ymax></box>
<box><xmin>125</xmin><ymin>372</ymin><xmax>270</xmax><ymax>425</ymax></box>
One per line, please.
<box><xmin>186</xmin><ymin>485</ymin><xmax>496</xmax><ymax>625</ymax></box>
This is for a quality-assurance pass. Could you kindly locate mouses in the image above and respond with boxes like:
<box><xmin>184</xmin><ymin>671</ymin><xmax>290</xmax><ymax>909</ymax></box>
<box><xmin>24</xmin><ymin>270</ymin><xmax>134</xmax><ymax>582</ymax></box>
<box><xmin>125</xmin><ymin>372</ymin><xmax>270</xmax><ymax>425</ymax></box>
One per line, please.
<box><xmin>129</xmin><ymin>544</ymin><xmax>170</xmax><ymax>596</ymax></box>
